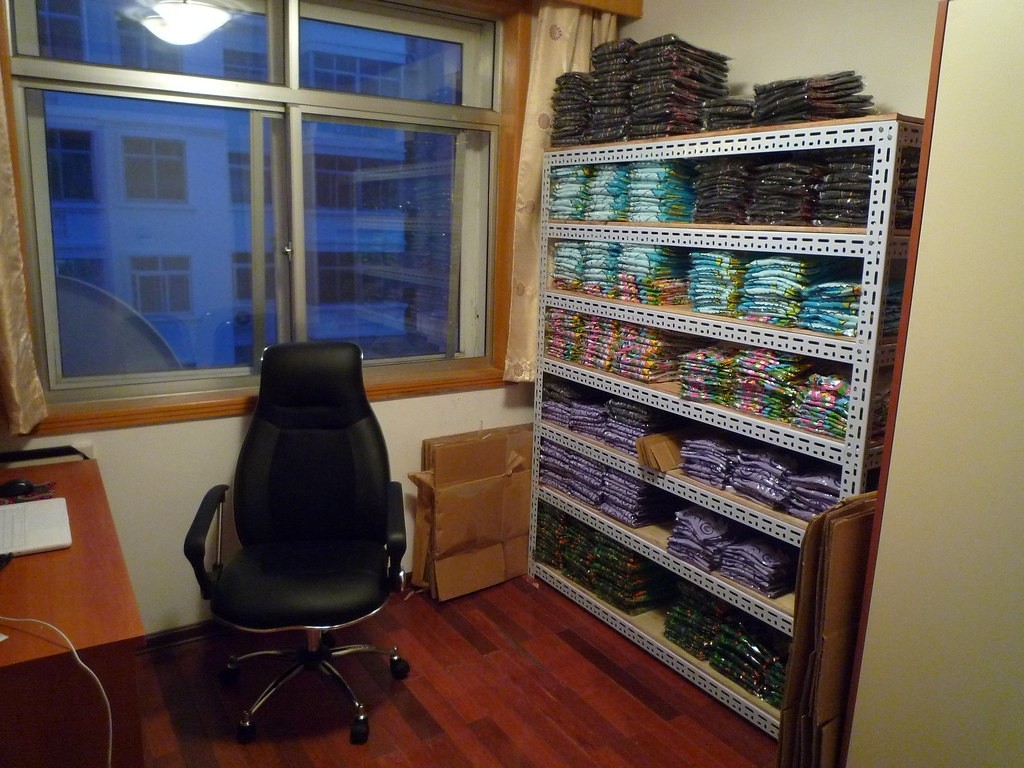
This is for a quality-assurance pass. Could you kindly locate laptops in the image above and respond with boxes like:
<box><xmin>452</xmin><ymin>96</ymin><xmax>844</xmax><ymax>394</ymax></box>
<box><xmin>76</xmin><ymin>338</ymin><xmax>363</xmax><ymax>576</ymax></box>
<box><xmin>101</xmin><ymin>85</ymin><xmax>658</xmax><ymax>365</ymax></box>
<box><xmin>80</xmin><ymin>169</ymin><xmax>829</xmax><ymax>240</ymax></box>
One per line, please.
<box><xmin>0</xmin><ymin>498</ymin><xmax>72</xmax><ymax>556</ymax></box>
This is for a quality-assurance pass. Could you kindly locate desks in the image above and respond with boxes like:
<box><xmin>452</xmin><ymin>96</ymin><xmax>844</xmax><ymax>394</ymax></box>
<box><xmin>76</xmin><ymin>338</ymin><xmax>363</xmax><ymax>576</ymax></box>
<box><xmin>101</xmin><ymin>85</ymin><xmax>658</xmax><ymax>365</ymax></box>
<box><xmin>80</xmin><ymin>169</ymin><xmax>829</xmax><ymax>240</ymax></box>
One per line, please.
<box><xmin>0</xmin><ymin>459</ymin><xmax>148</xmax><ymax>768</ymax></box>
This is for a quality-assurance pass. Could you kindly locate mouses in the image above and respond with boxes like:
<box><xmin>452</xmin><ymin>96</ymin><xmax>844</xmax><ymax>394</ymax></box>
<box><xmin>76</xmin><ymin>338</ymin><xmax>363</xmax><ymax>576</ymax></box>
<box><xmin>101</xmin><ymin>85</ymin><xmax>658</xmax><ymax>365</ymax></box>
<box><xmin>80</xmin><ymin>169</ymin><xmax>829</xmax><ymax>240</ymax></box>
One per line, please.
<box><xmin>0</xmin><ymin>479</ymin><xmax>34</xmax><ymax>498</ymax></box>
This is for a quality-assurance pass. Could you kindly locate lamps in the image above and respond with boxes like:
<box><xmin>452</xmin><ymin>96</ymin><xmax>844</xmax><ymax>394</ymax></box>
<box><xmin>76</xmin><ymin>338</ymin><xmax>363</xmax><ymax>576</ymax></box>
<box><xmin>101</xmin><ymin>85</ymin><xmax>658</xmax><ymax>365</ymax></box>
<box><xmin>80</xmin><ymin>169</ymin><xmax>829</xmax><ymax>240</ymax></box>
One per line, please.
<box><xmin>141</xmin><ymin>0</ymin><xmax>232</xmax><ymax>46</ymax></box>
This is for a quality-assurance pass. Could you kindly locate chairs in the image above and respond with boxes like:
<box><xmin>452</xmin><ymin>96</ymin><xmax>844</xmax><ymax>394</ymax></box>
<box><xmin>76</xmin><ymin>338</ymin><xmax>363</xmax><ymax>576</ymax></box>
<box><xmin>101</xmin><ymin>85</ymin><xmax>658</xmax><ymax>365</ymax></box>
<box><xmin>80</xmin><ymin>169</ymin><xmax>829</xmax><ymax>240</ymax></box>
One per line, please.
<box><xmin>183</xmin><ymin>342</ymin><xmax>410</xmax><ymax>744</ymax></box>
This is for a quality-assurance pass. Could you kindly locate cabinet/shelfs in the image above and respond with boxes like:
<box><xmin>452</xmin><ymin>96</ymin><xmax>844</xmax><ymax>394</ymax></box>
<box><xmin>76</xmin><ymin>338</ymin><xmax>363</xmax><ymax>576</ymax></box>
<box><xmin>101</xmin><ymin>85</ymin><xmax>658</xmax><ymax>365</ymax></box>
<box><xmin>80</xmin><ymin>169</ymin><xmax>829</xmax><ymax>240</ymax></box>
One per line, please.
<box><xmin>529</xmin><ymin>113</ymin><xmax>925</xmax><ymax>741</ymax></box>
